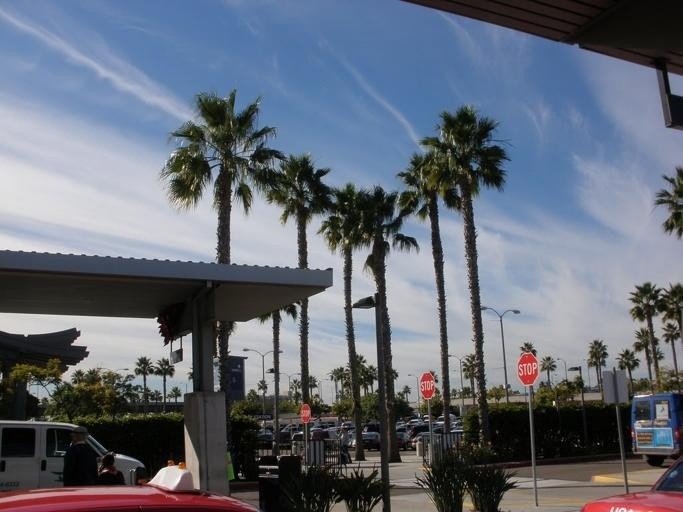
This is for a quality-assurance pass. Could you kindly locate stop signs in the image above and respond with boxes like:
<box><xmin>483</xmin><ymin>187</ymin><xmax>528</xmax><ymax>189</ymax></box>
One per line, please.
<box><xmin>300</xmin><ymin>404</ymin><xmax>311</xmax><ymax>423</ymax></box>
<box><xmin>419</xmin><ymin>372</ymin><xmax>435</xmax><ymax>400</ymax></box>
<box><xmin>517</xmin><ymin>352</ymin><xmax>539</xmax><ymax>386</ymax></box>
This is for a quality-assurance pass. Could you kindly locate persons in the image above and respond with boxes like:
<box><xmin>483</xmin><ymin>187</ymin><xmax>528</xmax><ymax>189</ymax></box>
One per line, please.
<box><xmin>62</xmin><ymin>425</ymin><xmax>98</xmax><ymax>486</ymax></box>
<box><xmin>97</xmin><ymin>451</ymin><xmax>125</xmax><ymax>485</ymax></box>
<box><xmin>336</xmin><ymin>425</ymin><xmax>352</xmax><ymax>463</ymax></box>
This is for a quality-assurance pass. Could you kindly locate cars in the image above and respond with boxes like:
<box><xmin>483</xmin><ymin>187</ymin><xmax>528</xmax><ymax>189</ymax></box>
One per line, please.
<box><xmin>0</xmin><ymin>461</ymin><xmax>257</xmax><ymax>511</ymax></box>
<box><xmin>580</xmin><ymin>454</ymin><xmax>683</xmax><ymax>512</ymax></box>
<box><xmin>249</xmin><ymin>413</ymin><xmax>465</xmax><ymax>450</ymax></box>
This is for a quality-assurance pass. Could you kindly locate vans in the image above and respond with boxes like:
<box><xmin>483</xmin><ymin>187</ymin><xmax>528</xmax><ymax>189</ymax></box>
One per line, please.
<box><xmin>0</xmin><ymin>420</ymin><xmax>146</xmax><ymax>491</ymax></box>
<box><xmin>630</xmin><ymin>392</ymin><xmax>683</xmax><ymax>466</ymax></box>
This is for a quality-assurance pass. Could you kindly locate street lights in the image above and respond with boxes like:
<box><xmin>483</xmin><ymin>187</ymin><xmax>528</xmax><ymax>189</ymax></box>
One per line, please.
<box><xmin>448</xmin><ymin>355</ymin><xmax>469</xmax><ymax>406</ymax></box>
<box><xmin>408</xmin><ymin>373</ymin><xmax>419</xmax><ymax>406</ymax></box>
<box><xmin>567</xmin><ymin>366</ymin><xmax>587</xmax><ymax>432</ymax></box>
<box><xmin>480</xmin><ymin>307</ymin><xmax>520</xmax><ymax>402</ymax></box>
<box><xmin>557</xmin><ymin>357</ymin><xmax>567</xmax><ymax>383</ymax></box>
<box><xmin>279</xmin><ymin>373</ymin><xmax>301</xmax><ymax>391</ymax></box>
<box><xmin>243</xmin><ymin>349</ymin><xmax>283</xmax><ymax>414</ymax></box>
<box><xmin>97</xmin><ymin>368</ymin><xmax>129</xmax><ymax>384</ymax></box>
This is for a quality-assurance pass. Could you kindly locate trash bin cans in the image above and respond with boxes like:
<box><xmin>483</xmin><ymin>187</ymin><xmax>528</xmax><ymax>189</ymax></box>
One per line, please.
<box><xmin>416</xmin><ymin>441</ymin><xmax>425</xmax><ymax>456</ymax></box>
<box><xmin>279</xmin><ymin>455</ymin><xmax>303</xmax><ymax>474</ymax></box>
<box><xmin>308</xmin><ymin>427</ymin><xmax>325</xmax><ymax>465</ymax></box>
<box><xmin>291</xmin><ymin>435</ymin><xmax>304</xmax><ymax>455</ymax></box>
